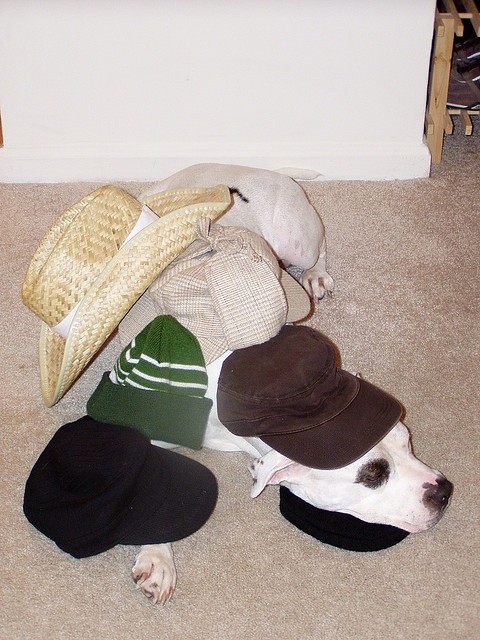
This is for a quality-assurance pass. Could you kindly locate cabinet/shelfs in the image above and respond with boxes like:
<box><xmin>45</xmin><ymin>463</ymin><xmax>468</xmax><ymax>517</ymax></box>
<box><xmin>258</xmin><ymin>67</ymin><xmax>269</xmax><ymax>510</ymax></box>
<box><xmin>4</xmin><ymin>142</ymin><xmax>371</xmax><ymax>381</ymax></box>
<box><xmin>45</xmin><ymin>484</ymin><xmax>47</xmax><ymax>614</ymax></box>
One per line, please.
<box><xmin>424</xmin><ymin>1</ymin><xmax>480</xmax><ymax>166</ymax></box>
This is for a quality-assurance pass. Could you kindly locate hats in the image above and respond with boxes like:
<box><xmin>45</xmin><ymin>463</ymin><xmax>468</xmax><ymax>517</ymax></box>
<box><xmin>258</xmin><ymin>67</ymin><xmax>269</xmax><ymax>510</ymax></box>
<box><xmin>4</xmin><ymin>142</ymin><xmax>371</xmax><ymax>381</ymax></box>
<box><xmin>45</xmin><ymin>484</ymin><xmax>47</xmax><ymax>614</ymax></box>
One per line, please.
<box><xmin>86</xmin><ymin>315</ymin><xmax>213</xmax><ymax>450</ymax></box>
<box><xmin>23</xmin><ymin>415</ymin><xmax>218</xmax><ymax>559</ymax></box>
<box><xmin>21</xmin><ymin>184</ymin><xmax>231</xmax><ymax>408</ymax></box>
<box><xmin>216</xmin><ymin>326</ymin><xmax>403</xmax><ymax>470</ymax></box>
<box><xmin>118</xmin><ymin>216</ymin><xmax>311</xmax><ymax>366</ymax></box>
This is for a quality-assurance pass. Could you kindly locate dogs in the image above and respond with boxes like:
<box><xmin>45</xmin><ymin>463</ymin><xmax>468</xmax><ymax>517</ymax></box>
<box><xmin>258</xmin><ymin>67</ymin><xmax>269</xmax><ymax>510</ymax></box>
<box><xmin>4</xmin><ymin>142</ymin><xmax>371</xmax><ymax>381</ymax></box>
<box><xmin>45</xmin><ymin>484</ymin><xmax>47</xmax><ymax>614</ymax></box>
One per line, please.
<box><xmin>119</xmin><ymin>161</ymin><xmax>455</xmax><ymax>607</ymax></box>
